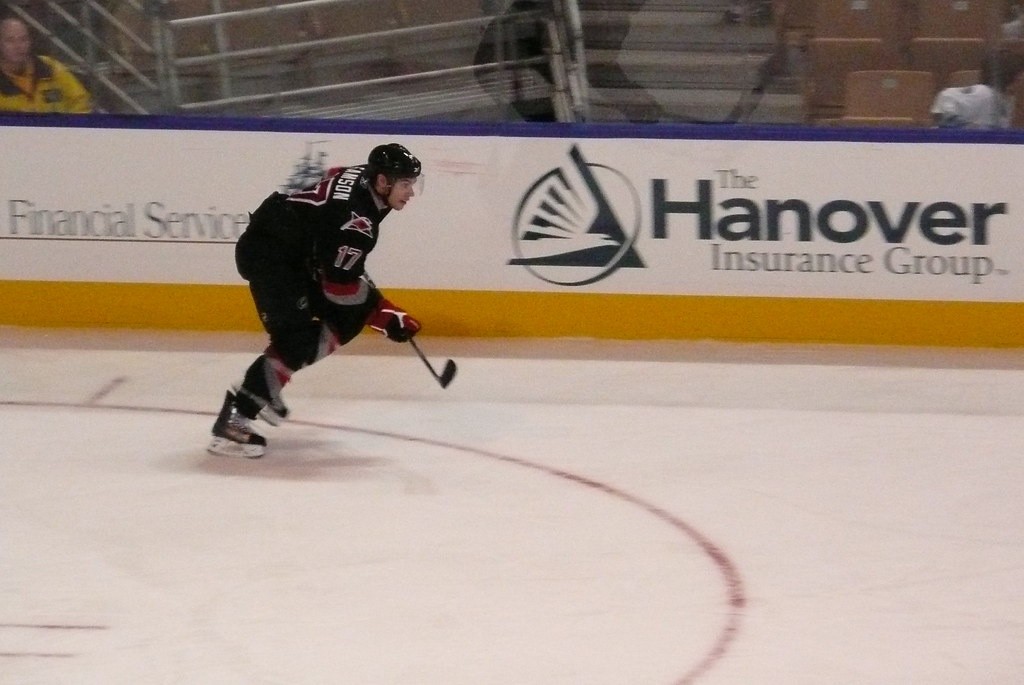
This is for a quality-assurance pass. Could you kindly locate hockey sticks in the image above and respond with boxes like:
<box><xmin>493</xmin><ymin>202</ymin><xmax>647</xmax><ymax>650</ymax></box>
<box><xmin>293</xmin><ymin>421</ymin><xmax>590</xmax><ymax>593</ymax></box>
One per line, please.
<box><xmin>362</xmin><ymin>270</ymin><xmax>458</xmax><ymax>390</ymax></box>
<box><xmin>585</xmin><ymin>83</ymin><xmax>766</xmax><ymax>125</ymax></box>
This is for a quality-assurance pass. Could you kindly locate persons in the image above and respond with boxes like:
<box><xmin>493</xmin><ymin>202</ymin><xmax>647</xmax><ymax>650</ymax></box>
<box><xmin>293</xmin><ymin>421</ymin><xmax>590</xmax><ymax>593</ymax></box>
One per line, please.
<box><xmin>932</xmin><ymin>40</ymin><xmax>1024</xmax><ymax>133</ymax></box>
<box><xmin>0</xmin><ymin>17</ymin><xmax>92</xmax><ymax>116</ymax></box>
<box><xmin>205</xmin><ymin>142</ymin><xmax>422</xmax><ymax>458</ymax></box>
<box><xmin>474</xmin><ymin>0</ymin><xmax>669</xmax><ymax>128</ymax></box>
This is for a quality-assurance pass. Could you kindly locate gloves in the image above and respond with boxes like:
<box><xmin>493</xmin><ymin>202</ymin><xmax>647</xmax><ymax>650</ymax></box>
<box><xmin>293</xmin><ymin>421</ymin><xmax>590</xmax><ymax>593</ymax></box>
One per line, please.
<box><xmin>366</xmin><ymin>297</ymin><xmax>422</xmax><ymax>344</ymax></box>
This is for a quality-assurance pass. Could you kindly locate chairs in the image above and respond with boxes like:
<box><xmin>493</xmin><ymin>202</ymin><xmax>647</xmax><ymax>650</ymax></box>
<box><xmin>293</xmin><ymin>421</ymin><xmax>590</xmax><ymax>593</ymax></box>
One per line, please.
<box><xmin>773</xmin><ymin>1</ymin><xmax>1024</xmax><ymax>132</ymax></box>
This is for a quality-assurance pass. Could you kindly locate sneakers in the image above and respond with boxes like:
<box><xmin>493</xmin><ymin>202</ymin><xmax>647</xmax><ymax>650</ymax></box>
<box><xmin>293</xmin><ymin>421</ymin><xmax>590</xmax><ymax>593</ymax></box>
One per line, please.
<box><xmin>230</xmin><ymin>364</ymin><xmax>290</xmax><ymax>428</ymax></box>
<box><xmin>207</xmin><ymin>389</ymin><xmax>266</xmax><ymax>458</ymax></box>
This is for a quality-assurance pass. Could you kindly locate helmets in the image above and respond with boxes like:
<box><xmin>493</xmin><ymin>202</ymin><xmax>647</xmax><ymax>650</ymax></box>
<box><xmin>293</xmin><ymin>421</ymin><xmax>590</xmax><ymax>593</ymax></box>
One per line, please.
<box><xmin>367</xmin><ymin>143</ymin><xmax>421</xmax><ymax>178</ymax></box>
